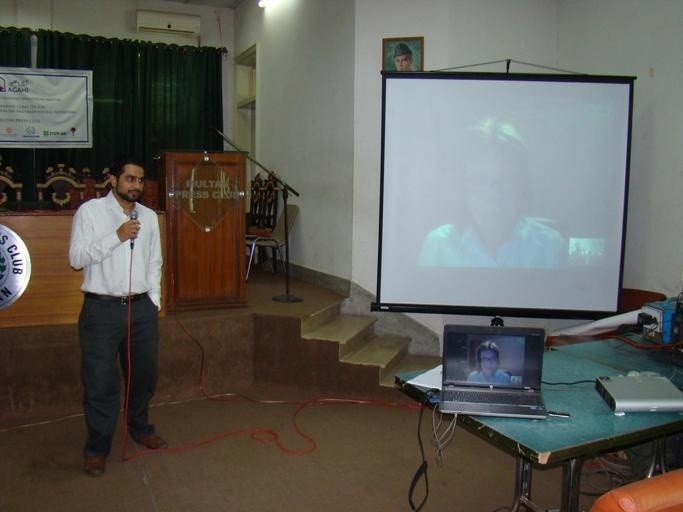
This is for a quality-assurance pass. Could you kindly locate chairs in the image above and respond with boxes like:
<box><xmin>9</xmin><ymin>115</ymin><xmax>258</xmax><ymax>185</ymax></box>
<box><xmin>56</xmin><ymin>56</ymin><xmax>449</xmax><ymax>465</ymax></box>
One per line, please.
<box><xmin>245</xmin><ymin>172</ymin><xmax>298</xmax><ymax>282</ymax></box>
<box><xmin>583</xmin><ymin>469</ymin><xmax>683</xmax><ymax>512</ymax></box>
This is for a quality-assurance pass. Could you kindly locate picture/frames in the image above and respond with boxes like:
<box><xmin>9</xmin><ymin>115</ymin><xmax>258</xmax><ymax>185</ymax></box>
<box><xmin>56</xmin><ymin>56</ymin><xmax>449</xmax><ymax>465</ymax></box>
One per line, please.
<box><xmin>381</xmin><ymin>36</ymin><xmax>424</xmax><ymax>72</ymax></box>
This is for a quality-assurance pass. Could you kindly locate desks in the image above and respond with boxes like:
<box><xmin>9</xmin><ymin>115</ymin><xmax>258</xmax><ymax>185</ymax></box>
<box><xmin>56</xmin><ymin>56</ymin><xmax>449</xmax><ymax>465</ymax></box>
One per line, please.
<box><xmin>551</xmin><ymin>336</ymin><xmax>682</xmax><ymax>390</ymax></box>
<box><xmin>395</xmin><ymin>350</ymin><xmax>683</xmax><ymax>512</ymax></box>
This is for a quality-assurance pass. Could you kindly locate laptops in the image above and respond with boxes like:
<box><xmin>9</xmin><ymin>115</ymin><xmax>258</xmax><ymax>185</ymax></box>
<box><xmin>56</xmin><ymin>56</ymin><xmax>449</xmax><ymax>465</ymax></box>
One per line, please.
<box><xmin>438</xmin><ymin>324</ymin><xmax>547</xmax><ymax>419</ymax></box>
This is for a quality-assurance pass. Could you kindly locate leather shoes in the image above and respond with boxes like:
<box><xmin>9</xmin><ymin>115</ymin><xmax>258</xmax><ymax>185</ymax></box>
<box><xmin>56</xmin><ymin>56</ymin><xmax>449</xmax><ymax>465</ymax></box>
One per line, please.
<box><xmin>85</xmin><ymin>455</ymin><xmax>105</xmax><ymax>477</ymax></box>
<box><xmin>139</xmin><ymin>434</ymin><xmax>168</xmax><ymax>450</ymax></box>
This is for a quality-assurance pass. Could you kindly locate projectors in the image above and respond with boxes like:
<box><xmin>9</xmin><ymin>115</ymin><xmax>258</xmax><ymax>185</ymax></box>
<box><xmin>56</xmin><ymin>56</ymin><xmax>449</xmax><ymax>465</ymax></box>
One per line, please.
<box><xmin>595</xmin><ymin>371</ymin><xmax>683</xmax><ymax>416</ymax></box>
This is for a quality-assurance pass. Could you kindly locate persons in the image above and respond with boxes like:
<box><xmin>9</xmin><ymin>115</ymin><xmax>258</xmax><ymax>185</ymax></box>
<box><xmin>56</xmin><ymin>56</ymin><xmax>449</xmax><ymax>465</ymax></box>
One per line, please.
<box><xmin>68</xmin><ymin>158</ymin><xmax>166</xmax><ymax>475</ymax></box>
<box><xmin>420</xmin><ymin>114</ymin><xmax>565</xmax><ymax>268</ymax></box>
<box><xmin>392</xmin><ymin>43</ymin><xmax>414</xmax><ymax>72</ymax></box>
<box><xmin>467</xmin><ymin>340</ymin><xmax>510</xmax><ymax>384</ymax></box>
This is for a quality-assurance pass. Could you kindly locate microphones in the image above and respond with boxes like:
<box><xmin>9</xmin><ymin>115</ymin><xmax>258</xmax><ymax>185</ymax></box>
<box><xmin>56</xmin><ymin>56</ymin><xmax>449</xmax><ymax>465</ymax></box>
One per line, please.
<box><xmin>129</xmin><ymin>210</ymin><xmax>138</xmax><ymax>250</ymax></box>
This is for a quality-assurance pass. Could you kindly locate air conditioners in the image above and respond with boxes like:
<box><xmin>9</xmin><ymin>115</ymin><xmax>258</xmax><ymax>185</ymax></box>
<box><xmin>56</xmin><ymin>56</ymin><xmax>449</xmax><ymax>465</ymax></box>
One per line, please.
<box><xmin>135</xmin><ymin>8</ymin><xmax>201</xmax><ymax>37</ymax></box>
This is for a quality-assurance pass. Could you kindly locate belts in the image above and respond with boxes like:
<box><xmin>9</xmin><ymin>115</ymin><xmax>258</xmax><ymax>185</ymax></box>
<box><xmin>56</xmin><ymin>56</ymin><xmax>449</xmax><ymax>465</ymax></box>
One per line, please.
<box><xmin>85</xmin><ymin>291</ymin><xmax>146</xmax><ymax>305</ymax></box>
